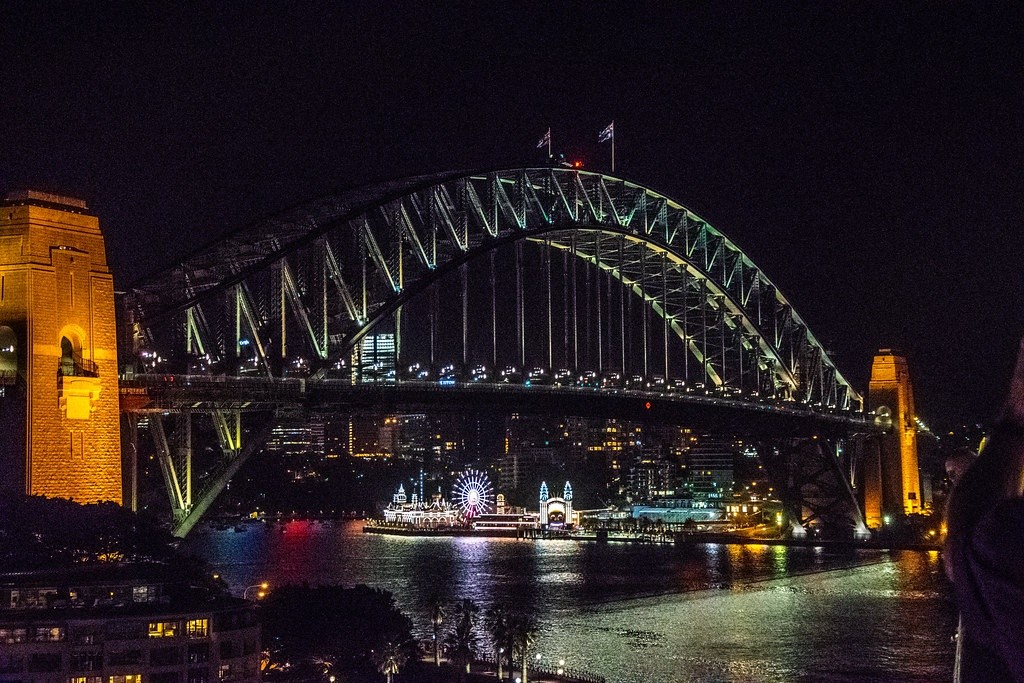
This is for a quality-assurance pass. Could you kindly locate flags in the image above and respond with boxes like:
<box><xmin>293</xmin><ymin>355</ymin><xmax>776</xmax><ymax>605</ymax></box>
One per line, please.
<box><xmin>536</xmin><ymin>131</ymin><xmax>549</xmax><ymax>148</ymax></box>
<box><xmin>598</xmin><ymin>124</ymin><xmax>612</xmax><ymax>143</ymax></box>
